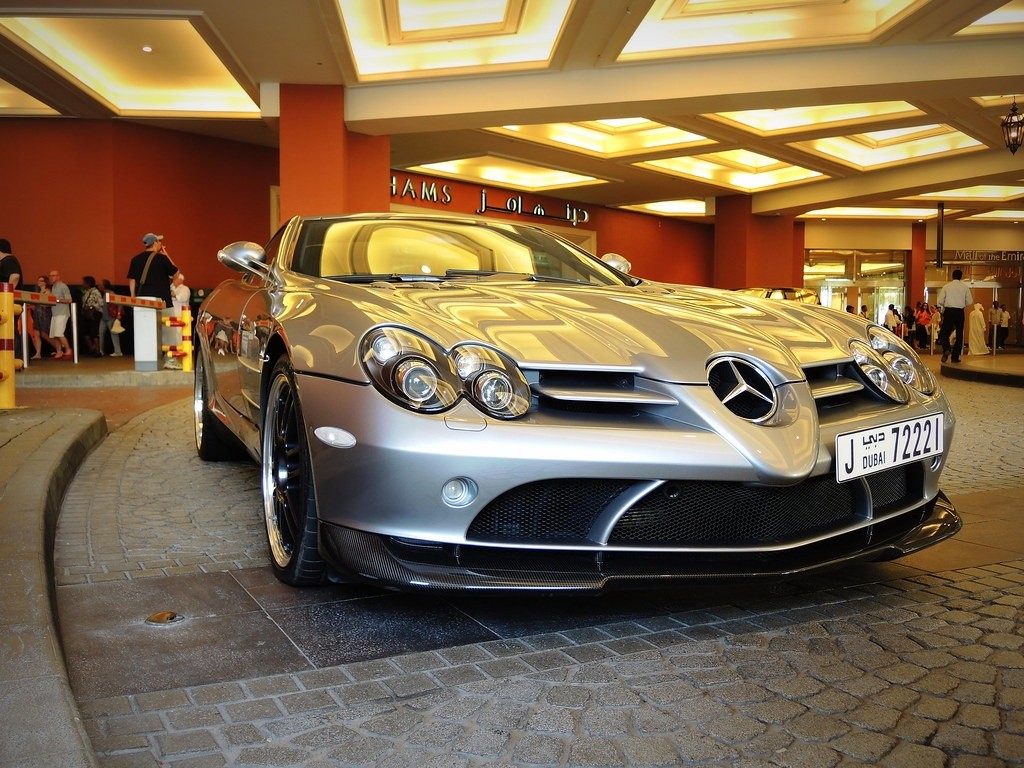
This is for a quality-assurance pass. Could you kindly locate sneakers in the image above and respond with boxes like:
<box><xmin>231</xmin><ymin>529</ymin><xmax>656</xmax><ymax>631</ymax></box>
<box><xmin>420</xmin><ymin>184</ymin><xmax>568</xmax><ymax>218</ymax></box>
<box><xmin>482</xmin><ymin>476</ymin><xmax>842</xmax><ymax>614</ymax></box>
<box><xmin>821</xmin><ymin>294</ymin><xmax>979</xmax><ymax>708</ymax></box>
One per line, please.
<box><xmin>164</xmin><ymin>358</ymin><xmax>183</xmax><ymax>369</ymax></box>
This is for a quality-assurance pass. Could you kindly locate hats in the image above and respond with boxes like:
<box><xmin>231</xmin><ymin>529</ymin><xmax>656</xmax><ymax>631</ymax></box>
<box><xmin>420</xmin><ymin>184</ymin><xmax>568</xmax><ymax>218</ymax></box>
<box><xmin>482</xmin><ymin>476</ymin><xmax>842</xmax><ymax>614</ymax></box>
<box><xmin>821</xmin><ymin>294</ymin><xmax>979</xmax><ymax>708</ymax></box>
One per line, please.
<box><xmin>143</xmin><ymin>233</ymin><xmax>163</xmax><ymax>246</ymax></box>
<box><xmin>169</xmin><ymin>274</ymin><xmax>185</xmax><ymax>280</ymax></box>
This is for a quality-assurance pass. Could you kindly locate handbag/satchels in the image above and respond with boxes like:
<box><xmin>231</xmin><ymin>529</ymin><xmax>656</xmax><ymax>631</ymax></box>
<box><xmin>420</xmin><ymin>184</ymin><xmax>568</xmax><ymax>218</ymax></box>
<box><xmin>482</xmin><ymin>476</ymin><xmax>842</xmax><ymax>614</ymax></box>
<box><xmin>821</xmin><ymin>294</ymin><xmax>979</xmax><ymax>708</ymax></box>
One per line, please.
<box><xmin>85</xmin><ymin>309</ymin><xmax>103</xmax><ymax>320</ymax></box>
<box><xmin>111</xmin><ymin>319</ymin><xmax>125</xmax><ymax>334</ymax></box>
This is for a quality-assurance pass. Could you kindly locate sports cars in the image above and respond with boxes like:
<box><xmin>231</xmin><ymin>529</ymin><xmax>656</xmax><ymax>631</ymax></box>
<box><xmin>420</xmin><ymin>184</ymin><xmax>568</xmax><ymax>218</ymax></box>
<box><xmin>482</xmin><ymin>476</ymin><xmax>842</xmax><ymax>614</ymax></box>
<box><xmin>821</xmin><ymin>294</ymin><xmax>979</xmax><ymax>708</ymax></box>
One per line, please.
<box><xmin>193</xmin><ymin>210</ymin><xmax>965</xmax><ymax>596</ymax></box>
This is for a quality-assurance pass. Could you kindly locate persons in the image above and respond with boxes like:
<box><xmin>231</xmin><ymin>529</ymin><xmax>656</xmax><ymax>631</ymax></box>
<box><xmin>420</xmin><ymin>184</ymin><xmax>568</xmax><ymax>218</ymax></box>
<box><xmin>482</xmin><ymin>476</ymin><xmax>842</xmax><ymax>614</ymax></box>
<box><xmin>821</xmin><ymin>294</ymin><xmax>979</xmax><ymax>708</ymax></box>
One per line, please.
<box><xmin>0</xmin><ymin>239</ymin><xmax>123</xmax><ymax>360</ymax></box>
<box><xmin>937</xmin><ymin>269</ymin><xmax>972</xmax><ymax>362</ymax></box>
<box><xmin>968</xmin><ymin>303</ymin><xmax>990</xmax><ymax>355</ymax></box>
<box><xmin>201</xmin><ymin>310</ymin><xmax>237</xmax><ymax>356</ymax></box>
<box><xmin>988</xmin><ymin>301</ymin><xmax>1012</xmax><ymax>349</ymax></box>
<box><xmin>885</xmin><ymin>301</ymin><xmax>943</xmax><ymax>351</ymax></box>
<box><xmin>846</xmin><ymin>305</ymin><xmax>869</xmax><ymax>320</ymax></box>
<box><xmin>127</xmin><ymin>233</ymin><xmax>190</xmax><ymax>361</ymax></box>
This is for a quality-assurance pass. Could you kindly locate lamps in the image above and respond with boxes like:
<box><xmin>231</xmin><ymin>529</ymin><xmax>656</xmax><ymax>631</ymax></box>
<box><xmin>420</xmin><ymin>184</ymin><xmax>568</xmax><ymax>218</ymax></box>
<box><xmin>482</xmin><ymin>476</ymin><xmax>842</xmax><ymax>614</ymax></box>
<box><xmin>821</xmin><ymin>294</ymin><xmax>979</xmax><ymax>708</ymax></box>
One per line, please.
<box><xmin>1000</xmin><ymin>96</ymin><xmax>1024</xmax><ymax>156</ymax></box>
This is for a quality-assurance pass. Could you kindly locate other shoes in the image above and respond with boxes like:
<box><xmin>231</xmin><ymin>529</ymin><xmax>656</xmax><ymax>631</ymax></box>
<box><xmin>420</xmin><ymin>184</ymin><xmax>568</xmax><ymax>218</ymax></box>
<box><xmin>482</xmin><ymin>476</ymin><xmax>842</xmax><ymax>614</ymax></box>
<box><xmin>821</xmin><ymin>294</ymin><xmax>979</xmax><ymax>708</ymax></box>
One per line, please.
<box><xmin>110</xmin><ymin>353</ymin><xmax>123</xmax><ymax>356</ymax></box>
<box><xmin>941</xmin><ymin>349</ymin><xmax>951</xmax><ymax>362</ymax></box>
<box><xmin>54</xmin><ymin>351</ymin><xmax>64</xmax><ymax>360</ymax></box>
<box><xmin>953</xmin><ymin>358</ymin><xmax>961</xmax><ymax>362</ymax></box>
<box><xmin>30</xmin><ymin>356</ymin><xmax>41</xmax><ymax>360</ymax></box>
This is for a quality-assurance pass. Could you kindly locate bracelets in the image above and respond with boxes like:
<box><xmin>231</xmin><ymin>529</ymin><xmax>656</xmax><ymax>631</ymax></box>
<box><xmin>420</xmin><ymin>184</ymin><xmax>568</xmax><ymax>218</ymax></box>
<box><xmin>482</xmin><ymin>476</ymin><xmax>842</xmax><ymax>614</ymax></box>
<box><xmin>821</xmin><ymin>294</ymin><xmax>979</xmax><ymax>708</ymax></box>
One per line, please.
<box><xmin>117</xmin><ymin>312</ymin><xmax>121</xmax><ymax>315</ymax></box>
<box><xmin>57</xmin><ymin>299</ymin><xmax>60</xmax><ymax>303</ymax></box>
